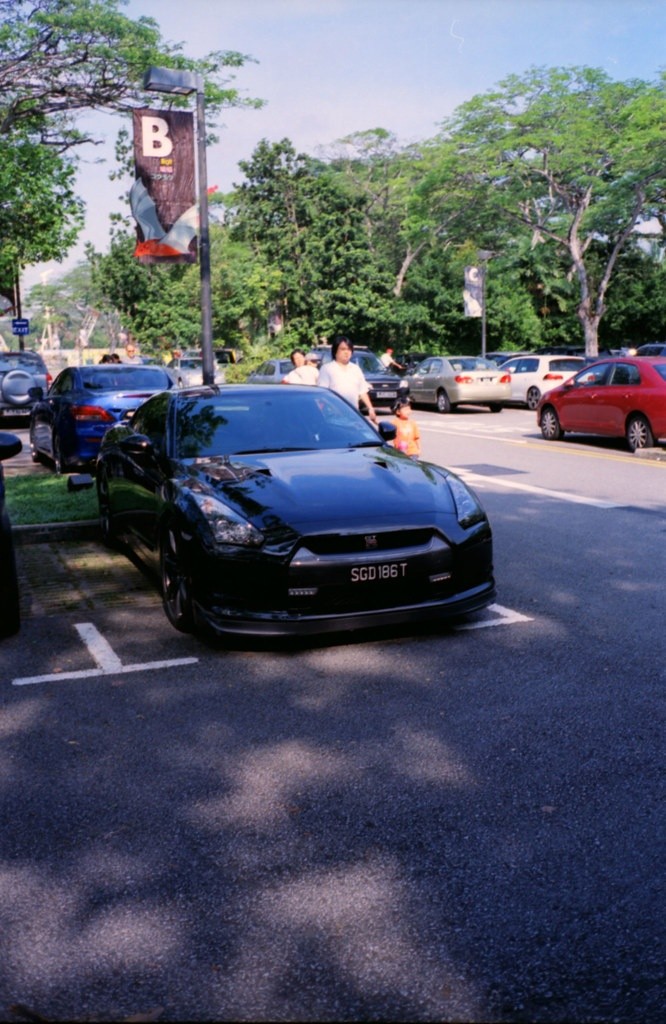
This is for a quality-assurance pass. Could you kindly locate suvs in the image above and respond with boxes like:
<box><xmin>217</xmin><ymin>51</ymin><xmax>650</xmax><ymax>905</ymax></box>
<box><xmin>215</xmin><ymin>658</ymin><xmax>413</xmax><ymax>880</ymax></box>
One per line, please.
<box><xmin>499</xmin><ymin>354</ymin><xmax>594</xmax><ymax>410</ymax></box>
<box><xmin>312</xmin><ymin>345</ymin><xmax>409</xmax><ymax>414</ymax></box>
<box><xmin>0</xmin><ymin>352</ymin><xmax>54</xmax><ymax>422</ymax></box>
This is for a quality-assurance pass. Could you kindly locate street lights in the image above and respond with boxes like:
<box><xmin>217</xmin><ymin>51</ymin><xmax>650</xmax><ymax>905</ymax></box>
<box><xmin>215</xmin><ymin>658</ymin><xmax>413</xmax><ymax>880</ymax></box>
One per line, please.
<box><xmin>144</xmin><ymin>65</ymin><xmax>216</xmax><ymax>385</ymax></box>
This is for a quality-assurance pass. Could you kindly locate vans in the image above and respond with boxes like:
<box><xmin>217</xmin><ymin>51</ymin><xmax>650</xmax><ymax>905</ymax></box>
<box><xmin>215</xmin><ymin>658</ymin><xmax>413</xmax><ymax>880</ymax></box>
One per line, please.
<box><xmin>535</xmin><ymin>346</ymin><xmax>612</xmax><ymax>370</ymax></box>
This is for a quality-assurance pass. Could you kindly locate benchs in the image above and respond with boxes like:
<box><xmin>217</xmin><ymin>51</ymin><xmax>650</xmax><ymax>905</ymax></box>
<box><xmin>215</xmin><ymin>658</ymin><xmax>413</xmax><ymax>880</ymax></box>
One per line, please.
<box><xmin>453</xmin><ymin>363</ymin><xmax>486</xmax><ymax>370</ymax></box>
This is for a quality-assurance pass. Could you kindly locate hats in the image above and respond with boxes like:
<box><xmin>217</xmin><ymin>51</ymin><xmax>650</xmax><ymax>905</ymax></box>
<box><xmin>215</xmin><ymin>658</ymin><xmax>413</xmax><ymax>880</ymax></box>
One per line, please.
<box><xmin>305</xmin><ymin>353</ymin><xmax>322</xmax><ymax>361</ymax></box>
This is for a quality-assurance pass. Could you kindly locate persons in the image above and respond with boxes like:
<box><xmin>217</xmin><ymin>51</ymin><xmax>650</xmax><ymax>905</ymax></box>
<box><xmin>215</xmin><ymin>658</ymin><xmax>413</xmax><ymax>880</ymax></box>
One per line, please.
<box><xmin>281</xmin><ymin>337</ymin><xmax>421</xmax><ymax>460</ymax></box>
<box><xmin>99</xmin><ymin>344</ymin><xmax>183</xmax><ymax>368</ymax></box>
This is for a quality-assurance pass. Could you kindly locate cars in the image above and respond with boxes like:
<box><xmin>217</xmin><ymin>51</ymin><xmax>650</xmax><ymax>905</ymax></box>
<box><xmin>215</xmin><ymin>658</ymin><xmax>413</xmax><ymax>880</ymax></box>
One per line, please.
<box><xmin>95</xmin><ymin>382</ymin><xmax>497</xmax><ymax>641</ymax></box>
<box><xmin>247</xmin><ymin>359</ymin><xmax>295</xmax><ymax>384</ymax></box>
<box><xmin>404</xmin><ymin>356</ymin><xmax>512</xmax><ymax>414</ymax></box>
<box><xmin>537</xmin><ymin>356</ymin><xmax>666</xmax><ymax>452</ymax></box>
<box><xmin>482</xmin><ymin>352</ymin><xmax>516</xmax><ymax>368</ymax></box>
<box><xmin>166</xmin><ymin>356</ymin><xmax>225</xmax><ymax>388</ymax></box>
<box><xmin>27</xmin><ymin>365</ymin><xmax>182</xmax><ymax>476</ymax></box>
<box><xmin>633</xmin><ymin>344</ymin><xmax>666</xmax><ymax>358</ymax></box>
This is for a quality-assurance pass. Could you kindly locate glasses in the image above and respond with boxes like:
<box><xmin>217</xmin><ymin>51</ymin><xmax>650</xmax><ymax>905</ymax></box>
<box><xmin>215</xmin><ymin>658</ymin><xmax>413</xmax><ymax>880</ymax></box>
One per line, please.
<box><xmin>128</xmin><ymin>350</ymin><xmax>135</xmax><ymax>352</ymax></box>
